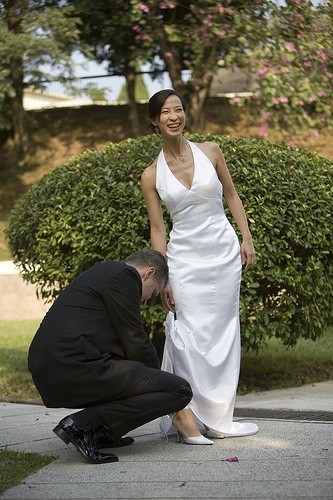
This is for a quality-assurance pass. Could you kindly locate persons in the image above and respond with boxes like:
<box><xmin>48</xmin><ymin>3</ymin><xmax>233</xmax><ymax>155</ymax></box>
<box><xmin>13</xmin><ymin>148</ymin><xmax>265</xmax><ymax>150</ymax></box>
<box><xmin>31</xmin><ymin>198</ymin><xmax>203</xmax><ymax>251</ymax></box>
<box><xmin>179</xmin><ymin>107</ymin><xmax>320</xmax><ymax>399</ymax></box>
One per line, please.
<box><xmin>28</xmin><ymin>250</ymin><xmax>193</xmax><ymax>464</ymax></box>
<box><xmin>141</xmin><ymin>90</ymin><xmax>259</xmax><ymax>444</ymax></box>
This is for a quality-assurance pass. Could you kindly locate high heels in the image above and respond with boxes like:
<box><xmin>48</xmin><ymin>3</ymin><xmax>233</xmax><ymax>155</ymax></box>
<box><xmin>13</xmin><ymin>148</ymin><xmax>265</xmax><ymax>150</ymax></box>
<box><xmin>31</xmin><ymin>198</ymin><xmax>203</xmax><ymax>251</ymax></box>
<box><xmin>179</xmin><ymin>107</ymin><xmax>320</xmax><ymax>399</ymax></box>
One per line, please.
<box><xmin>171</xmin><ymin>409</ymin><xmax>224</xmax><ymax>445</ymax></box>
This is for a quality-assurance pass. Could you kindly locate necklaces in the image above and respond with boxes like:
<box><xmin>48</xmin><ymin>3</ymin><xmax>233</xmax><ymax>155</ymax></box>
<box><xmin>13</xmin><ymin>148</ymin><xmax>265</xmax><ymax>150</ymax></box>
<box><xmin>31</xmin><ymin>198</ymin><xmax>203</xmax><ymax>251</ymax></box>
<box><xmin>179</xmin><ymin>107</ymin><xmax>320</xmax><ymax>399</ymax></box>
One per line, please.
<box><xmin>164</xmin><ymin>141</ymin><xmax>186</xmax><ymax>161</ymax></box>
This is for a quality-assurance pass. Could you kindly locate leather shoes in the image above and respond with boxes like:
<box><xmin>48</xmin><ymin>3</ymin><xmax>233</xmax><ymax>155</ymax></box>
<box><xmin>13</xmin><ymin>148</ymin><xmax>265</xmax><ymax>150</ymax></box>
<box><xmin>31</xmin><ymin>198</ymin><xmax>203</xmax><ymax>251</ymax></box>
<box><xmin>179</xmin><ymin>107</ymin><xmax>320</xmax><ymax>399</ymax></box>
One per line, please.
<box><xmin>52</xmin><ymin>415</ymin><xmax>134</xmax><ymax>464</ymax></box>
